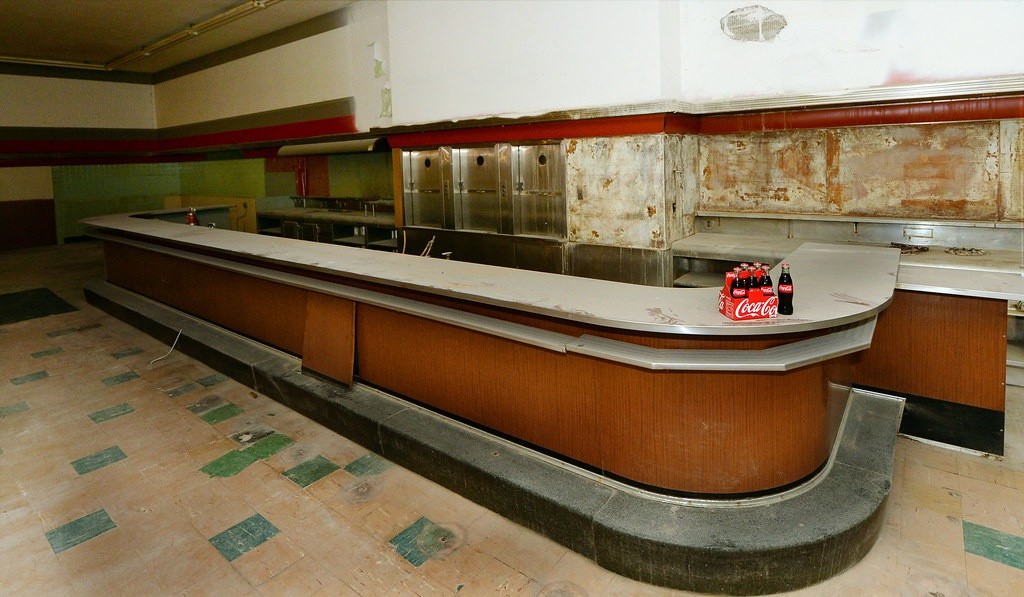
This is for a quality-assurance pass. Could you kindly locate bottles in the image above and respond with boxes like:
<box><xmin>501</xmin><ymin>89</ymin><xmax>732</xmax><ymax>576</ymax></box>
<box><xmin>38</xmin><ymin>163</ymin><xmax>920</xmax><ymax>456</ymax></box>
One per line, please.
<box><xmin>778</xmin><ymin>264</ymin><xmax>793</xmax><ymax>315</ymax></box>
<box><xmin>740</xmin><ymin>264</ymin><xmax>748</xmax><ymax>271</ymax></box>
<box><xmin>731</xmin><ymin>267</ymin><xmax>745</xmax><ymax>298</ymax></box>
<box><xmin>745</xmin><ymin>266</ymin><xmax>760</xmax><ymax>297</ymax></box>
<box><xmin>759</xmin><ymin>266</ymin><xmax>773</xmax><ymax>296</ymax></box>
<box><xmin>753</xmin><ymin>262</ymin><xmax>761</xmax><ymax>270</ymax></box>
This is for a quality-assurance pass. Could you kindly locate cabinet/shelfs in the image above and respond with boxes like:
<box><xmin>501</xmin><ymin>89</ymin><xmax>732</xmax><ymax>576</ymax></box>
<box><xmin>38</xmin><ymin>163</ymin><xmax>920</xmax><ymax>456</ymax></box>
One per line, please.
<box><xmin>256</xmin><ymin>206</ymin><xmax>397</xmax><ymax>251</ymax></box>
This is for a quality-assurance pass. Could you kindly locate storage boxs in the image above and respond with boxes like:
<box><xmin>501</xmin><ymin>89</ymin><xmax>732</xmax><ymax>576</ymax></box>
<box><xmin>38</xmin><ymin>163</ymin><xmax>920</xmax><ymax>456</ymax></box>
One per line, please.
<box><xmin>718</xmin><ymin>270</ymin><xmax>778</xmax><ymax>320</ymax></box>
